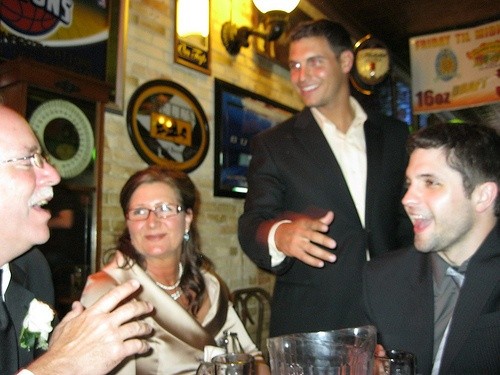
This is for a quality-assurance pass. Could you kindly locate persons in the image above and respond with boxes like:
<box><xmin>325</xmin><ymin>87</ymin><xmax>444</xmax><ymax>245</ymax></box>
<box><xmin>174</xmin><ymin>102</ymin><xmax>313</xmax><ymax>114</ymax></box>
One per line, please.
<box><xmin>80</xmin><ymin>166</ymin><xmax>270</xmax><ymax>375</ymax></box>
<box><xmin>0</xmin><ymin>103</ymin><xmax>153</xmax><ymax>375</ymax></box>
<box><xmin>238</xmin><ymin>18</ymin><xmax>414</xmax><ymax>375</ymax></box>
<box><xmin>340</xmin><ymin>122</ymin><xmax>500</xmax><ymax>375</ymax></box>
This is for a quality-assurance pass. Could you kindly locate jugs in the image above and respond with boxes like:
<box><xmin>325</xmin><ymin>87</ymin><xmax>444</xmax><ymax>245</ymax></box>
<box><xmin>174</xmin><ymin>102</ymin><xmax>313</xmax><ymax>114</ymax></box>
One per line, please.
<box><xmin>265</xmin><ymin>324</ymin><xmax>378</xmax><ymax>375</ymax></box>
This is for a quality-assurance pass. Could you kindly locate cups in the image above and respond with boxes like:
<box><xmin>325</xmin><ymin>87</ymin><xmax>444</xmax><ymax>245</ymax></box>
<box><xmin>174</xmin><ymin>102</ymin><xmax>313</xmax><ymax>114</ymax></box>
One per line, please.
<box><xmin>196</xmin><ymin>351</ymin><xmax>256</xmax><ymax>375</ymax></box>
<box><xmin>373</xmin><ymin>347</ymin><xmax>418</xmax><ymax>375</ymax></box>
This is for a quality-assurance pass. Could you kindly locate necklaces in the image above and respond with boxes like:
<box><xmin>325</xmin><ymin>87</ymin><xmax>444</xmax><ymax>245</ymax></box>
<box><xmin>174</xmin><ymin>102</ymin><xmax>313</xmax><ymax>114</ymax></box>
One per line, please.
<box><xmin>157</xmin><ymin>262</ymin><xmax>183</xmax><ymax>301</ymax></box>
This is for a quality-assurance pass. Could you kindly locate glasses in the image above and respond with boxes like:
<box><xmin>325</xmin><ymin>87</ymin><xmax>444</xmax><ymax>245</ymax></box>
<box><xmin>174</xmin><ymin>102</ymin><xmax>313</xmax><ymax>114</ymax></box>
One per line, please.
<box><xmin>125</xmin><ymin>204</ymin><xmax>186</xmax><ymax>221</ymax></box>
<box><xmin>3</xmin><ymin>150</ymin><xmax>49</xmax><ymax>169</ymax></box>
